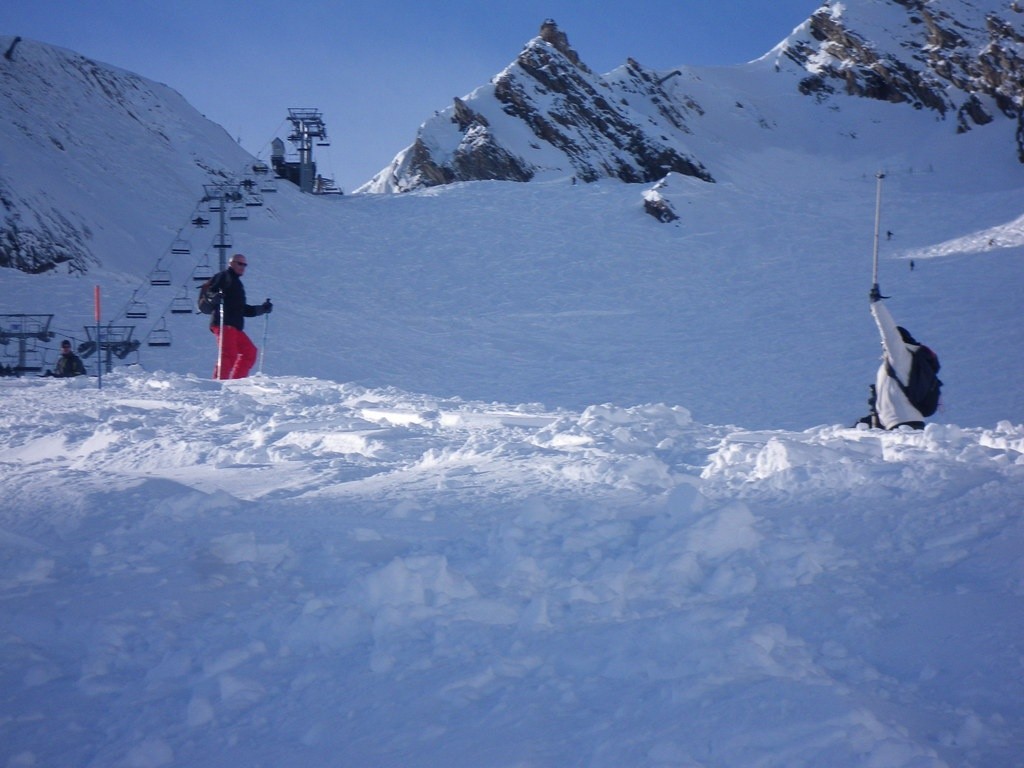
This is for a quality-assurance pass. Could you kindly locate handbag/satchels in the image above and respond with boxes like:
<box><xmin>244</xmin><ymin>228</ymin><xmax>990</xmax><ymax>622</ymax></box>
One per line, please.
<box><xmin>196</xmin><ymin>270</ymin><xmax>232</xmax><ymax>314</ymax></box>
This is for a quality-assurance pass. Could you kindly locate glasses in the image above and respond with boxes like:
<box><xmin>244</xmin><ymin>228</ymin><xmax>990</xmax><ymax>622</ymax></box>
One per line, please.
<box><xmin>232</xmin><ymin>261</ymin><xmax>248</xmax><ymax>267</ymax></box>
<box><xmin>62</xmin><ymin>345</ymin><xmax>71</xmax><ymax>348</ymax></box>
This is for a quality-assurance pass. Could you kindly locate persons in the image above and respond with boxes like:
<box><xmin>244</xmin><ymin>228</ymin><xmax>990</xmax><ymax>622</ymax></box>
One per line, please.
<box><xmin>53</xmin><ymin>340</ymin><xmax>87</xmax><ymax>378</ymax></box>
<box><xmin>197</xmin><ymin>254</ymin><xmax>273</xmax><ymax>380</ymax></box>
<box><xmin>847</xmin><ymin>289</ymin><xmax>925</xmax><ymax>430</ymax></box>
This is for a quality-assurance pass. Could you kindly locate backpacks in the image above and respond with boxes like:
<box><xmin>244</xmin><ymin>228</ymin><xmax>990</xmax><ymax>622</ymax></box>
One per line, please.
<box><xmin>884</xmin><ymin>343</ymin><xmax>943</xmax><ymax>417</ymax></box>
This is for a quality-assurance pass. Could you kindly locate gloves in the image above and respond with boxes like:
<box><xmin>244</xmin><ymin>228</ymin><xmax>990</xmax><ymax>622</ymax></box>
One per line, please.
<box><xmin>868</xmin><ymin>288</ymin><xmax>883</xmax><ymax>304</ymax></box>
<box><xmin>208</xmin><ymin>292</ymin><xmax>225</xmax><ymax>305</ymax></box>
<box><xmin>255</xmin><ymin>301</ymin><xmax>273</xmax><ymax>315</ymax></box>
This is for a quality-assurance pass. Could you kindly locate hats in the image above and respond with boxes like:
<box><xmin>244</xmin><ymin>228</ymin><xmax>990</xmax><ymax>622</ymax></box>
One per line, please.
<box><xmin>60</xmin><ymin>340</ymin><xmax>71</xmax><ymax>346</ymax></box>
<box><xmin>896</xmin><ymin>326</ymin><xmax>912</xmax><ymax>344</ymax></box>
